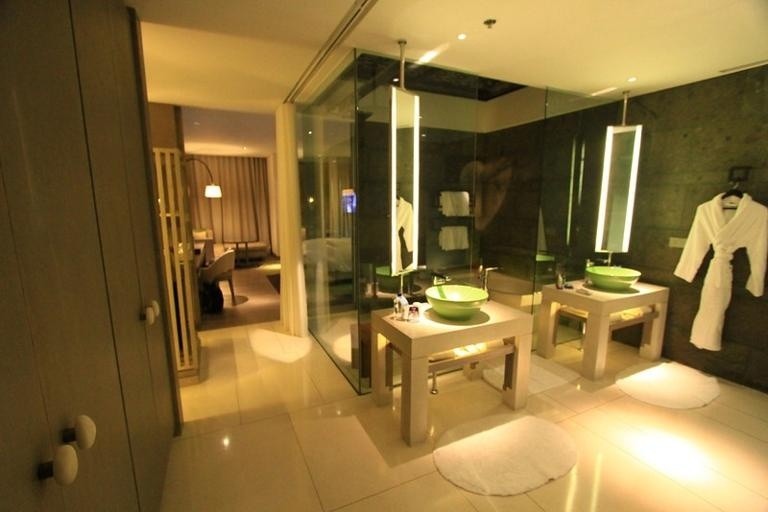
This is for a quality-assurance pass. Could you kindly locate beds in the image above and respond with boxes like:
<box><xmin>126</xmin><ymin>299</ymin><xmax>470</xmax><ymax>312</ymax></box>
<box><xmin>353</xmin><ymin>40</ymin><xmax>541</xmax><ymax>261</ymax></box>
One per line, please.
<box><xmin>302</xmin><ymin>237</ymin><xmax>353</xmax><ymax>273</ymax></box>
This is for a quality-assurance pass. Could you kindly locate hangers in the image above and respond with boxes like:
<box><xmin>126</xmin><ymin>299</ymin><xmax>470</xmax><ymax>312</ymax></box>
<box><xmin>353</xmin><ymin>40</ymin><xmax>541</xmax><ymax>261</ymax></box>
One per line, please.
<box><xmin>722</xmin><ymin>177</ymin><xmax>744</xmax><ymax>209</ymax></box>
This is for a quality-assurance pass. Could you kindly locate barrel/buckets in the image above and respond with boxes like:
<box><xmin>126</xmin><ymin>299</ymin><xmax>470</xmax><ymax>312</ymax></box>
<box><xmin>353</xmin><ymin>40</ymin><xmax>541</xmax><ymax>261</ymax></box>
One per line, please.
<box><xmin>393</xmin><ymin>292</ymin><xmax>409</xmax><ymax>321</ymax></box>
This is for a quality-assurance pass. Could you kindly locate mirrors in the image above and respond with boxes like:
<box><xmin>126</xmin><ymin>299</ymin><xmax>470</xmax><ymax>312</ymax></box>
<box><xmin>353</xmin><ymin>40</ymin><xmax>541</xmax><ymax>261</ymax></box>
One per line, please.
<box><xmin>594</xmin><ymin>124</ymin><xmax>643</xmax><ymax>254</ymax></box>
<box><xmin>390</xmin><ymin>85</ymin><xmax>419</xmax><ymax>276</ymax></box>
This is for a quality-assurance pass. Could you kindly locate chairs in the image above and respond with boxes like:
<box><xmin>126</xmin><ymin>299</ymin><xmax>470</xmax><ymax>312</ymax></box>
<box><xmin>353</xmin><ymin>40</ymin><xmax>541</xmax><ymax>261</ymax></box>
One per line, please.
<box><xmin>198</xmin><ymin>250</ymin><xmax>237</xmax><ymax>305</ymax></box>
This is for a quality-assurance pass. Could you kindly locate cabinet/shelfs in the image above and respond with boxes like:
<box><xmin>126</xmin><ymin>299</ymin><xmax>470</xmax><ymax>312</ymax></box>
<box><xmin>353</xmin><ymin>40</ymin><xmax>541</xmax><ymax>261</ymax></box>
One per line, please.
<box><xmin>0</xmin><ymin>0</ymin><xmax>183</xmax><ymax>512</ymax></box>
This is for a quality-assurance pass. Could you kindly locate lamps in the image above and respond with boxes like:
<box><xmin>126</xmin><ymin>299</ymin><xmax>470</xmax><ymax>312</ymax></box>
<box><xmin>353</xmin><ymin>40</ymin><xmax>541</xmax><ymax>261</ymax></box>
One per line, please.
<box><xmin>186</xmin><ymin>158</ymin><xmax>224</xmax><ymax>199</ymax></box>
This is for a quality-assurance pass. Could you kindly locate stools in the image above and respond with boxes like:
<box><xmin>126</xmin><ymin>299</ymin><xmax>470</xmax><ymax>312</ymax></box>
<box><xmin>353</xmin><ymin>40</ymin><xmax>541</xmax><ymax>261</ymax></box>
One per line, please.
<box><xmin>224</xmin><ymin>241</ymin><xmax>269</xmax><ymax>261</ymax></box>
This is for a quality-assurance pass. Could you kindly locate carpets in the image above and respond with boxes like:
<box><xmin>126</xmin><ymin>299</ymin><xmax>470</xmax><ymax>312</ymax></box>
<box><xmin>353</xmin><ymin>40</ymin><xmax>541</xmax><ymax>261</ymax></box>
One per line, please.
<box><xmin>481</xmin><ymin>338</ymin><xmax>663</xmax><ymax>425</ymax></box>
<box><xmin>614</xmin><ymin>360</ymin><xmax>720</xmax><ymax>410</ymax></box>
<box><xmin>433</xmin><ymin>413</ymin><xmax>577</xmax><ymax>496</ymax></box>
<box><xmin>194</xmin><ymin>266</ymin><xmax>281</xmax><ymax>332</ymax></box>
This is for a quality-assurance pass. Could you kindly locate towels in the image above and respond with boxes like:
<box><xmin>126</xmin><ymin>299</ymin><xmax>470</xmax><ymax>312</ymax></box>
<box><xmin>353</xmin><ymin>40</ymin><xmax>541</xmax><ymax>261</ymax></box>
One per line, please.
<box><xmin>440</xmin><ymin>190</ymin><xmax>471</xmax><ymax>217</ymax></box>
<box><xmin>438</xmin><ymin>227</ymin><xmax>469</xmax><ymax>251</ymax></box>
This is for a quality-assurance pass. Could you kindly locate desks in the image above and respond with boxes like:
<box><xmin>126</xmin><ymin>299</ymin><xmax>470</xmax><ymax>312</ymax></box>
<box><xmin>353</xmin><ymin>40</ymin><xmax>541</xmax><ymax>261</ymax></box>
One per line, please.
<box><xmin>536</xmin><ymin>277</ymin><xmax>670</xmax><ymax>382</ymax></box>
<box><xmin>370</xmin><ymin>301</ymin><xmax>534</xmax><ymax>446</ymax></box>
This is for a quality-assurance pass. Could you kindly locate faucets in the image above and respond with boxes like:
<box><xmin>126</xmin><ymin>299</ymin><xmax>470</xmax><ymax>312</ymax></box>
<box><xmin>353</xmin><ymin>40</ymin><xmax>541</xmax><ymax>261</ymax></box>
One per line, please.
<box><xmin>406</xmin><ymin>264</ymin><xmax>427</xmax><ymax>294</ymax></box>
<box><xmin>584</xmin><ymin>257</ymin><xmax>609</xmax><ymax>268</ymax></box>
<box><xmin>477</xmin><ymin>264</ymin><xmax>504</xmax><ymax>292</ymax></box>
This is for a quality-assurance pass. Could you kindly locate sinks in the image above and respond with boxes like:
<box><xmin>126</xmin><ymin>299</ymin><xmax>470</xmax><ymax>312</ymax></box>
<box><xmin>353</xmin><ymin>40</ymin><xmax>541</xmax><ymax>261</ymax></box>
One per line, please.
<box><xmin>425</xmin><ymin>285</ymin><xmax>488</xmax><ymax>321</ymax></box>
<box><xmin>376</xmin><ymin>265</ymin><xmax>427</xmax><ymax>290</ymax></box>
<box><xmin>431</xmin><ymin>271</ymin><xmax>452</xmax><ymax>285</ymax></box>
<box><xmin>585</xmin><ymin>265</ymin><xmax>642</xmax><ymax>292</ymax></box>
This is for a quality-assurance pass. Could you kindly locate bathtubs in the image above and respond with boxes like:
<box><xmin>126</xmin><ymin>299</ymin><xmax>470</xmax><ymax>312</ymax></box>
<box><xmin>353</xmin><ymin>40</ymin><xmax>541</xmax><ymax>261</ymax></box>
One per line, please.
<box><xmin>442</xmin><ymin>270</ymin><xmax>545</xmax><ymax>309</ymax></box>
